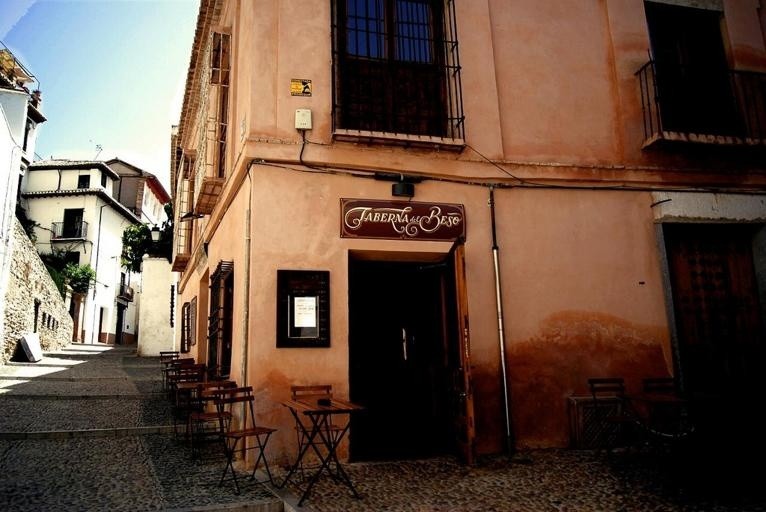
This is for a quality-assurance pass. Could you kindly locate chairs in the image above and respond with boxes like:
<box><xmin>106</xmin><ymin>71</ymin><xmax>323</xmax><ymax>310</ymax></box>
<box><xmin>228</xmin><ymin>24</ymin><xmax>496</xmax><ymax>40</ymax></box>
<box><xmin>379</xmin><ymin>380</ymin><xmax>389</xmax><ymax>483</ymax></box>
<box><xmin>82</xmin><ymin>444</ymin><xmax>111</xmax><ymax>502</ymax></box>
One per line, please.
<box><xmin>588</xmin><ymin>379</ymin><xmax>643</xmax><ymax>492</ymax></box>
<box><xmin>160</xmin><ymin>352</ymin><xmax>345</xmax><ymax>507</ymax></box>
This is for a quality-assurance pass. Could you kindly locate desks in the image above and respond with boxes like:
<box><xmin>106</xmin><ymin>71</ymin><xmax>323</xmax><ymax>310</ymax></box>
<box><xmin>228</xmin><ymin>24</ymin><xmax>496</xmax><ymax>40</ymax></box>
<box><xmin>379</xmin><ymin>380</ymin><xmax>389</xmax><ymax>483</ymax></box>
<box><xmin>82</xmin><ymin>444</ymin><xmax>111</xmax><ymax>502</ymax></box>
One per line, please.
<box><xmin>279</xmin><ymin>400</ymin><xmax>364</xmax><ymax>508</ymax></box>
